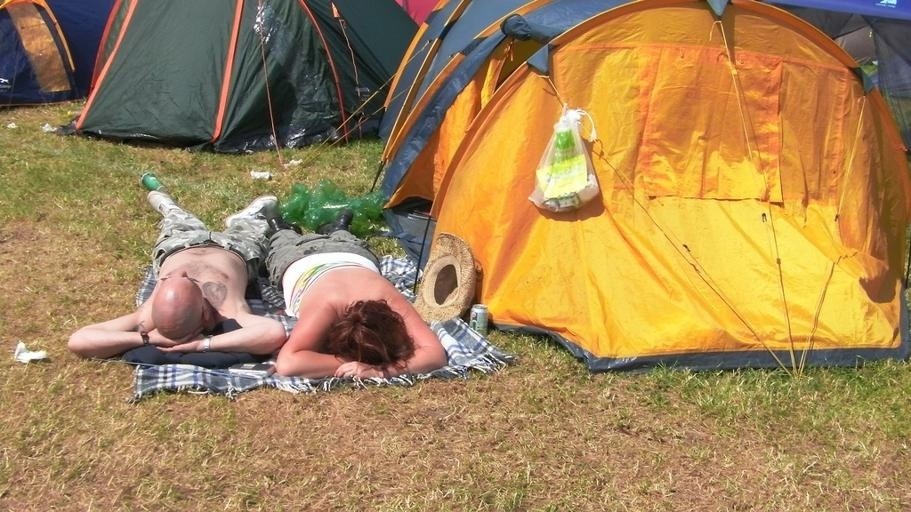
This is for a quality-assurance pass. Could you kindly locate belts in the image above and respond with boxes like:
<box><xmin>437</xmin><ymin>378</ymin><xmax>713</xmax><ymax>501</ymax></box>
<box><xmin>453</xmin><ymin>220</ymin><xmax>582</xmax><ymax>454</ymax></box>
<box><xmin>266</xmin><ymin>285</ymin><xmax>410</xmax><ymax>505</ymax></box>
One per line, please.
<box><xmin>160</xmin><ymin>242</ymin><xmax>244</xmax><ymax>266</ymax></box>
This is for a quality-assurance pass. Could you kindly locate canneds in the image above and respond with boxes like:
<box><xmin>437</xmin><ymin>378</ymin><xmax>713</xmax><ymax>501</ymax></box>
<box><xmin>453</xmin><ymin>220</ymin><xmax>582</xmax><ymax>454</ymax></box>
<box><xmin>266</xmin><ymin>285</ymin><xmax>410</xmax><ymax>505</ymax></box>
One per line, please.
<box><xmin>469</xmin><ymin>304</ymin><xmax>488</xmax><ymax>336</ymax></box>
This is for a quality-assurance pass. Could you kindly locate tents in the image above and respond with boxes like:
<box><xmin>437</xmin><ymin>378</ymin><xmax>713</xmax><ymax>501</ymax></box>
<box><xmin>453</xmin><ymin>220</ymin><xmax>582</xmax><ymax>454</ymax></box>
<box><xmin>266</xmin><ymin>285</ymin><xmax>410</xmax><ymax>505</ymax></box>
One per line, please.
<box><xmin>372</xmin><ymin>1</ymin><xmax>911</xmax><ymax>378</ymax></box>
<box><xmin>0</xmin><ymin>0</ymin><xmax>117</xmax><ymax>106</ymax></box>
<box><xmin>55</xmin><ymin>0</ymin><xmax>423</xmax><ymax>156</ymax></box>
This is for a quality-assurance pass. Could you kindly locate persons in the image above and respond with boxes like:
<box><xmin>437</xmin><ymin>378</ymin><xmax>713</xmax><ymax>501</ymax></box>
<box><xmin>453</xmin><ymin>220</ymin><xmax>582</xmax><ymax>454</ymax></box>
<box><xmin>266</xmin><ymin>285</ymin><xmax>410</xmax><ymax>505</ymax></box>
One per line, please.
<box><xmin>262</xmin><ymin>209</ymin><xmax>448</xmax><ymax>381</ymax></box>
<box><xmin>67</xmin><ymin>191</ymin><xmax>288</xmax><ymax>358</ymax></box>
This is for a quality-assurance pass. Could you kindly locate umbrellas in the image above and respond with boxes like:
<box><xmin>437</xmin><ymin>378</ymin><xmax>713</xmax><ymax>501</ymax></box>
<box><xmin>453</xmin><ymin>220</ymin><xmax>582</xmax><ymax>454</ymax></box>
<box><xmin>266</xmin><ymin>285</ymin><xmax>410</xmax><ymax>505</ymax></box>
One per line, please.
<box><xmin>764</xmin><ymin>1</ymin><xmax>911</xmax><ymax>104</ymax></box>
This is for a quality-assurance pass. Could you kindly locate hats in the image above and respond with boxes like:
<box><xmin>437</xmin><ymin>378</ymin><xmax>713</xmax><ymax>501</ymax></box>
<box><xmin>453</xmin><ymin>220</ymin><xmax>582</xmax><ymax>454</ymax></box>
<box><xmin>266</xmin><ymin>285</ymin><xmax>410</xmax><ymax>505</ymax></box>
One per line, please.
<box><xmin>415</xmin><ymin>232</ymin><xmax>476</xmax><ymax>320</ymax></box>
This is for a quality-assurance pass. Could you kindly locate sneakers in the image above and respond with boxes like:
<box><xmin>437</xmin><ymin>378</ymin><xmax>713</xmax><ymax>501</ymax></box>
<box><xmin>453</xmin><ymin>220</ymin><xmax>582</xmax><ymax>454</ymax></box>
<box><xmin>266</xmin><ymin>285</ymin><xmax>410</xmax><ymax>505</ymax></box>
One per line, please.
<box><xmin>226</xmin><ymin>195</ymin><xmax>301</xmax><ymax>233</ymax></box>
<box><xmin>147</xmin><ymin>191</ymin><xmax>182</xmax><ymax>216</ymax></box>
<box><xmin>316</xmin><ymin>210</ymin><xmax>352</xmax><ymax>234</ymax></box>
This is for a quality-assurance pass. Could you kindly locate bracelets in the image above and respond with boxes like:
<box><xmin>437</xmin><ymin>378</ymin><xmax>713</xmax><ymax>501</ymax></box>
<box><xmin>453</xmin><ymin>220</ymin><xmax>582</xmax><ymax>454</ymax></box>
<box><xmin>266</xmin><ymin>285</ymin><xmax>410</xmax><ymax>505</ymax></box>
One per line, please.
<box><xmin>203</xmin><ymin>333</ymin><xmax>214</xmax><ymax>352</ymax></box>
<box><xmin>139</xmin><ymin>329</ymin><xmax>150</xmax><ymax>348</ymax></box>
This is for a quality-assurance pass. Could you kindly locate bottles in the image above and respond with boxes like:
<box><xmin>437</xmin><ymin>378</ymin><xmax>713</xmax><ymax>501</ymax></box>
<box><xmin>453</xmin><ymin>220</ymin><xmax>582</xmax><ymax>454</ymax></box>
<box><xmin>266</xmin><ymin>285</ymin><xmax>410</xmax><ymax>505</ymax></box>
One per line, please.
<box><xmin>277</xmin><ymin>177</ymin><xmax>388</xmax><ymax>238</ymax></box>
<box><xmin>141</xmin><ymin>171</ymin><xmax>168</xmax><ymax>195</ymax></box>
